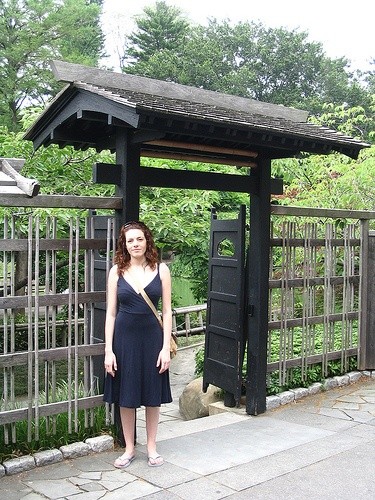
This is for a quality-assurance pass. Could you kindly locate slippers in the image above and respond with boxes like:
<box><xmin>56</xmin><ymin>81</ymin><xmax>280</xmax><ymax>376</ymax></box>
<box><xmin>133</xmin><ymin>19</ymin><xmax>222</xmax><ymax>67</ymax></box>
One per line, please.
<box><xmin>147</xmin><ymin>455</ymin><xmax>164</xmax><ymax>467</ymax></box>
<box><xmin>114</xmin><ymin>455</ymin><xmax>136</xmax><ymax>468</ymax></box>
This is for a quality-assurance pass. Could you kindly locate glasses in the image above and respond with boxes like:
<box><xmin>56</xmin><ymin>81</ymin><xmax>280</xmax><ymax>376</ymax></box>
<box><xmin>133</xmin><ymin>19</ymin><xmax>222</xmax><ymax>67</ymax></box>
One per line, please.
<box><xmin>124</xmin><ymin>221</ymin><xmax>141</xmax><ymax>227</ymax></box>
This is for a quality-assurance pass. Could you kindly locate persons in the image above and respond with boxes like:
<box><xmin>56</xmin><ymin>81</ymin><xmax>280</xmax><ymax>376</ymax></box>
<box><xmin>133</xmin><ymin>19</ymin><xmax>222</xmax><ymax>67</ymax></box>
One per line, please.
<box><xmin>103</xmin><ymin>221</ymin><xmax>173</xmax><ymax>469</ymax></box>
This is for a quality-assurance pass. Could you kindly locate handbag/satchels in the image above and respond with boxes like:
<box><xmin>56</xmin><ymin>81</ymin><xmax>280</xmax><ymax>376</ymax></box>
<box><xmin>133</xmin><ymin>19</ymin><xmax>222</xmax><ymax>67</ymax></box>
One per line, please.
<box><xmin>170</xmin><ymin>332</ymin><xmax>178</xmax><ymax>359</ymax></box>
